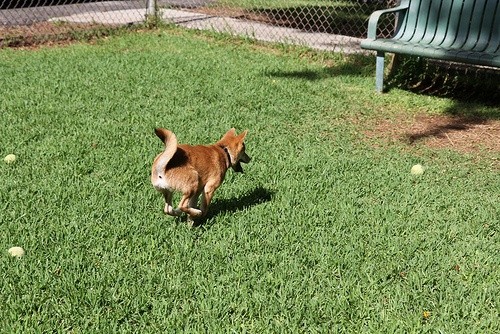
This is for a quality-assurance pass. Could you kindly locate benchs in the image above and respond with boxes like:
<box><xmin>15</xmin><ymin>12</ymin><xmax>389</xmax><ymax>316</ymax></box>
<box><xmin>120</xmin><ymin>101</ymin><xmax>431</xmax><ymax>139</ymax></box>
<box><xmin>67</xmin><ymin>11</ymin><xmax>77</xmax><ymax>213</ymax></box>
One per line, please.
<box><xmin>359</xmin><ymin>0</ymin><xmax>500</xmax><ymax>93</ymax></box>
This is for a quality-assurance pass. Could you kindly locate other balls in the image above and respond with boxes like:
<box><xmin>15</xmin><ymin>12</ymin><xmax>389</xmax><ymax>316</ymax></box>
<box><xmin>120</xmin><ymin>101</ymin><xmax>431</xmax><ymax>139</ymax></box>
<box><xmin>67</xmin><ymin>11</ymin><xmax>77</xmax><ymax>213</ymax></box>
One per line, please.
<box><xmin>5</xmin><ymin>151</ymin><xmax>18</xmax><ymax>165</ymax></box>
<box><xmin>8</xmin><ymin>246</ymin><xmax>26</xmax><ymax>258</ymax></box>
<box><xmin>411</xmin><ymin>164</ymin><xmax>423</xmax><ymax>175</ymax></box>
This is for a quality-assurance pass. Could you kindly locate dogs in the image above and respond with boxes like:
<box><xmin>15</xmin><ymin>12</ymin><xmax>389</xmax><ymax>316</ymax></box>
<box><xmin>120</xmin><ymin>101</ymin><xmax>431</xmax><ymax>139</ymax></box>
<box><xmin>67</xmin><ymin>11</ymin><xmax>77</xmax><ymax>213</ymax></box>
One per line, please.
<box><xmin>151</xmin><ymin>128</ymin><xmax>251</xmax><ymax>222</ymax></box>
